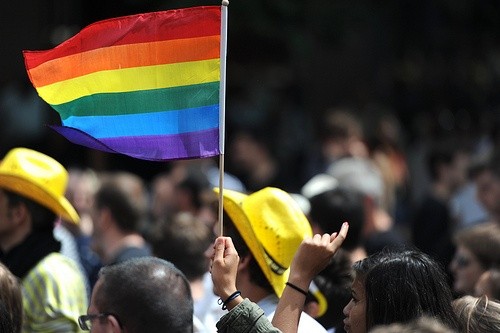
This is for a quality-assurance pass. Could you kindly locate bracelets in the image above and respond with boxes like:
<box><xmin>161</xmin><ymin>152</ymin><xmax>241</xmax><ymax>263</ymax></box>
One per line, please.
<box><xmin>218</xmin><ymin>291</ymin><xmax>241</xmax><ymax>310</ymax></box>
<box><xmin>286</xmin><ymin>282</ymin><xmax>307</xmax><ymax>295</ymax></box>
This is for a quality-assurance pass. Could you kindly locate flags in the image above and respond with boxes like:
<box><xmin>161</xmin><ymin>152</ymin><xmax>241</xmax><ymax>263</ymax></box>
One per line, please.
<box><xmin>23</xmin><ymin>5</ymin><xmax>222</xmax><ymax>161</ymax></box>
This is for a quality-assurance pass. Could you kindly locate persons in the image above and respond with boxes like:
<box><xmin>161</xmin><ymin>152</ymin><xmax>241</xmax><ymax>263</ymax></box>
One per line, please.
<box><xmin>206</xmin><ymin>186</ymin><xmax>327</xmax><ymax>333</ymax></box>
<box><xmin>88</xmin><ymin>258</ymin><xmax>194</xmax><ymax>333</ymax></box>
<box><xmin>0</xmin><ymin>148</ymin><xmax>87</xmax><ymax>333</ymax></box>
<box><xmin>0</xmin><ymin>107</ymin><xmax>500</xmax><ymax>333</ymax></box>
<box><xmin>271</xmin><ymin>221</ymin><xmax>455</xmax><ymax>333</ymax></box>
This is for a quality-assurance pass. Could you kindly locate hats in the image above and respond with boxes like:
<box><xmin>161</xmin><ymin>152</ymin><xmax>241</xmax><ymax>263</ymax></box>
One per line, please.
<box><xmin>0</xmin><ymin>146</ymin><xmax>81</xmax><ymax>224</ymax></box>
<box><xmin>212</xmin><ymin>186</ymin><xmax>328</xmax><ymax>318</ymax></box>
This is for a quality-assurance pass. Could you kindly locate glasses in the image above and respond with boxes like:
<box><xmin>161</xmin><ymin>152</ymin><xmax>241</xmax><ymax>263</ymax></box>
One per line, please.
<box><xmin>78</xmin><ymin>314</ymin><xmax>123</xmax><ymax>331</ymax></box>
